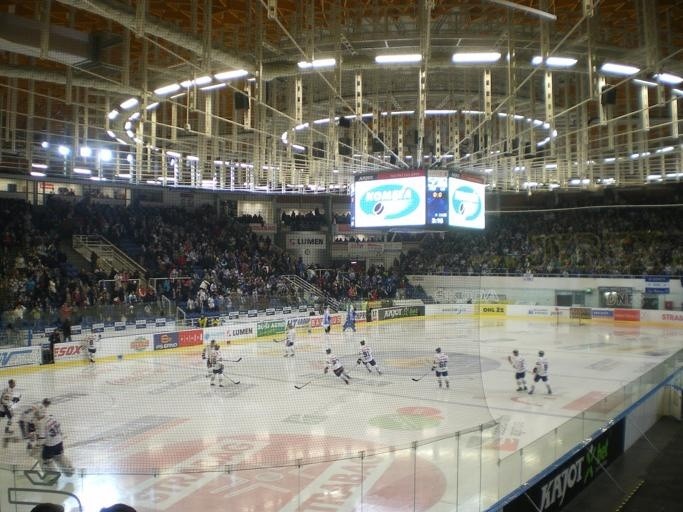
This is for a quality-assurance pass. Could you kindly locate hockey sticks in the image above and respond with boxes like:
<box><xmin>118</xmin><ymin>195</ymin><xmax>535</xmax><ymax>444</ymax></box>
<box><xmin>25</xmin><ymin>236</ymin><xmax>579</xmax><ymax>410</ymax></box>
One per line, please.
<box><xmin>507</xmin><ymin>355</ymin><xmax>534</xmax><ymax>375</ymax></box>
<box><xmin>273</xmin><ymin>338</ymin><xmax>288</xmax><ymax>342</ymax></box>
<box><xmin>412</xmin><ymin>371</ymin><xmax>433</xmax><ymax>381</ymax></box>
<box><xmin>224</xmin><ymin>374</ymin><xmax>240</xmax><ymax>384</ymax></box>
<box><xmin>294</xmin><ymin>373</ymin><xmax>325</xmax><ymax>389</ymax></box>
<box><xmin>223</xmin><ymin>358</ymin><xmax>242</xmax><ymax>362</ymax></box>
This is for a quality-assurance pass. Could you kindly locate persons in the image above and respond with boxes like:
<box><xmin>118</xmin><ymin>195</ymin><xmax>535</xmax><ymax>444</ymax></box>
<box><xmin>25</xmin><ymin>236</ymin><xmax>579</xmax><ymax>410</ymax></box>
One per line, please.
<box><xmin>86</xmin><ymin>340</ymin><xmax>96</xmax><ymax>369</ymax></box>
<box><xmin>0</xmin><ymin>378</ymin><xmax>21</xmax><ymax>434</ymax></box>
<box><xmin>322</xmin><ymin>346</ymin><xmax>351</xmax><ymax>385</ymax></box>
<box><xmin>19</xmin><ymin>397</ymin><xmax>50</xmax><ymax>449</ymax></box>
<box><xmin>208</xmin><ymin>344</ymin><xmax>225</xmax><ymax>386</ymax></box>
<box><xmin>429</xmin><ymin>347</ymin><xmax>450</xmax><ymax>388</ymax></box>
<box><xmin>356</xmin><ymin>340</ymin><xmax>383</xmax><ymax>375</ymax></box>
<box><xmin>32</xmin><ymin>408</ymin><xmax>75</xmax><ymax>472</ymax></box>
<box><xmin>281</xmin><ymin>323</ymin><xmax>295</xmax><ymax>356</ymax></box>
<box><xmin>530</xmin><ymin>350</ymin><xmax>552</xmax><ymax>388</ymax></box>
<box><xmin>0</xmin><ymin>184</ymin><xmax>429</xmax><ymax>356</ymax></box>
<box><xmin>428</xmin><ymin>186</ymin><xmax>682</xmax><ymax>283</ymax></box>
<box><xmin>202</xmin><ymin>339</ymin><xmax>216</xmax><ymax>378</ymax></box>
<box><xmin>506</xmin><ymin>348</ymin><xmax>528</xmax><ymax>391</ymax></box>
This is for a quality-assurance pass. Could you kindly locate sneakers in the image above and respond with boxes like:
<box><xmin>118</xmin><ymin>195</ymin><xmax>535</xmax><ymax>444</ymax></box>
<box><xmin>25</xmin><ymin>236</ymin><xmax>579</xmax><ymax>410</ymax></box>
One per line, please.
<box><xmin>517</xmin><ymin>386</ymin><xmax>533</xmax><ymax>394</ymax></box>
<box><xmin>345</xmin><ymin>375</ymin><xmax>349</xmax><ymax>384</ymax></box>
<box><xmin>439</xmin><ymin>383</ymin><xmax>449</xmax><ymax>387</ymax></box>
<box><xmin>211</xmin><ymin>384</ymin><xmax>223</xmax><ymax>387</ymax></box>
<box><xmin>548</xmin><ymin>389</ymin><xmax>551</xmax><ymax>393</ymax></box>
<box><xmin>283</xmin><ymin>354</ymin><xmax>294</xmax><ymax>357</ymax></box>
<box><xmin>368</xmin><ymin>370</ymin><xmax>382</xmax><ymax>375</ymax></box>
